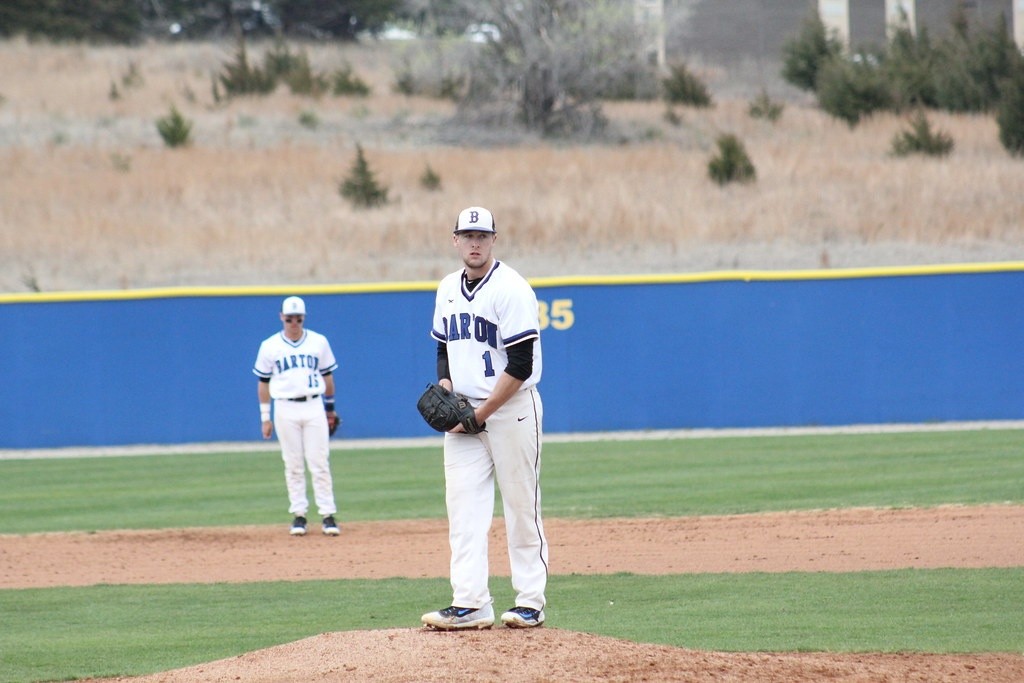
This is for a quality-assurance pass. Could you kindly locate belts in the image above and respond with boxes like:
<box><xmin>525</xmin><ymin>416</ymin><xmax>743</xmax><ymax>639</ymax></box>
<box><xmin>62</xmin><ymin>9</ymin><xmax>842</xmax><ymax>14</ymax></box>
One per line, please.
<box><xmin>288</xmin><ymin>394</ymin><xmax>318</xmax><ymax>401</ymax></box>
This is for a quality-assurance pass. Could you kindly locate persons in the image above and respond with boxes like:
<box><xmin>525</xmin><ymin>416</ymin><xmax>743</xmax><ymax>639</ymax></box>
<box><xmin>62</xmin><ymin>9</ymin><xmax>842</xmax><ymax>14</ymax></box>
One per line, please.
<box><xmin>417</xmin><ymin>207</ymin><xmax>548</xmax><ymax>628</ymax></box>
<box><xmin>252</xmin><ymin>296</ymin><xmax>339</xmax><ymax>535</ymax></box>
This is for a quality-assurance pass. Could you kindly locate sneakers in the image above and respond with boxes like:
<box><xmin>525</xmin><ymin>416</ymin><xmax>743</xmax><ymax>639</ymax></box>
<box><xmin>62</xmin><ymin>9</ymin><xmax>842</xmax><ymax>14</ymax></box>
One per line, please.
<box><xmin>322</xmin><ymin>516</ymin><xmax>340</xmax><ymax>535</ymax></box>
<box><xmin>500</xmin><ymin>605</ymin><xmax>545</xmax><ymax>628</ymax></box>
<box><xmin>421</xmin><ymin>596</ymin><xmax>495</xmax><ymax>631</ymax></box>
<box><xmin>289</xmin><ymin>516</ymin><xmax>307</xmax><ymax>535</ymax></box>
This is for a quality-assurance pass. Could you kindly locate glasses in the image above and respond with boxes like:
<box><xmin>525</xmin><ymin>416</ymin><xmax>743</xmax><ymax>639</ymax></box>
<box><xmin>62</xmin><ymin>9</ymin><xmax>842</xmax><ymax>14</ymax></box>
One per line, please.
<box><xmin>284</xmin><ymin>318</ymin><xmax>303</xmax><ymax>323</ymax></box>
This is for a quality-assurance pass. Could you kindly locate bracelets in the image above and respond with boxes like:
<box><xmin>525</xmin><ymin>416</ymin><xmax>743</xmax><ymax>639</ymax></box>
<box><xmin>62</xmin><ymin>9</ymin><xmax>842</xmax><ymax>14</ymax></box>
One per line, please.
<box><xmin>259</xmin><ymin>403</ymin><xmax>271</xmax><ymax>422</ymax></box>
<box><xmin>324</xmin><ymin>395</ymin><xmax>335</xmax><ymax>412</ymax></box>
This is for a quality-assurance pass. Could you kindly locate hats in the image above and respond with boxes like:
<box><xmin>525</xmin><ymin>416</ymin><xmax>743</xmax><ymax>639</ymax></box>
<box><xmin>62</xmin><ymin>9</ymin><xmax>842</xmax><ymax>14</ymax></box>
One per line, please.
<box><xmin>282</xmin><ymin>296</ymin><xmax>305</xmax><ymax>315</ymax></box>
<box><xmin>453</xmin><ymin>206</ymin><xmax>497</xmax><ymax>233</ymax></box>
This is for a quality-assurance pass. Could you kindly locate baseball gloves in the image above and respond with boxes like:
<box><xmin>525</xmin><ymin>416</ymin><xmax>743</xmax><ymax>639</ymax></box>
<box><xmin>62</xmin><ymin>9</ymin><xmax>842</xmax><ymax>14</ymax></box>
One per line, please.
<box><xmin>416</xmin><ymin>382</ymin><xmax>488</xmax><ymax>435</ymax></box>
<box><xmin>327</xmin><ymin>414</ymin><xmax>340</xmax><ymax>436</ymax></box>
